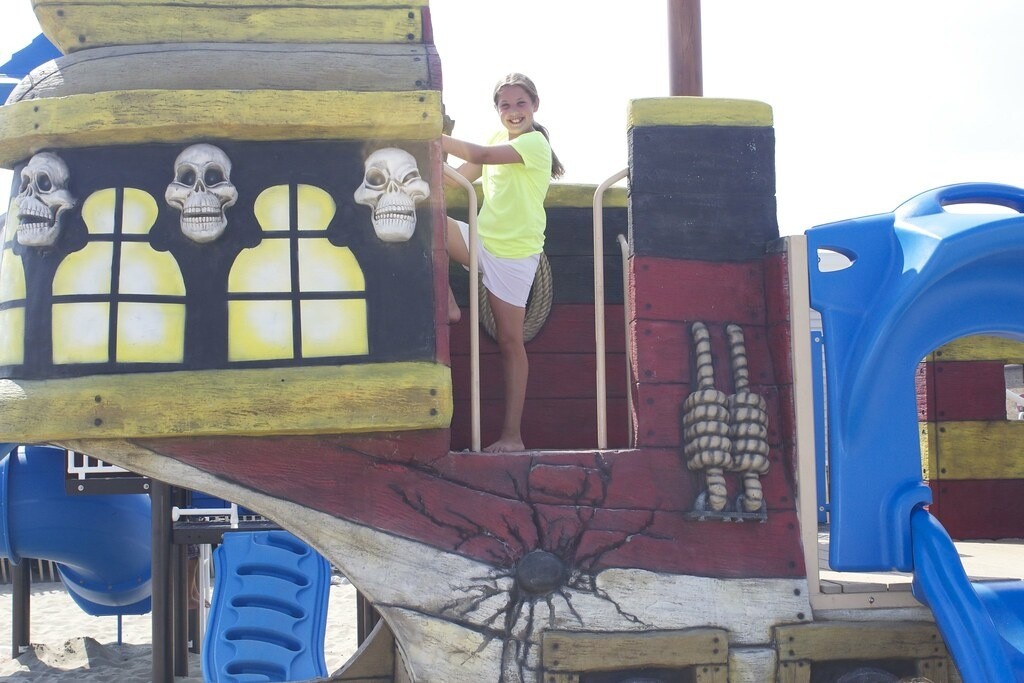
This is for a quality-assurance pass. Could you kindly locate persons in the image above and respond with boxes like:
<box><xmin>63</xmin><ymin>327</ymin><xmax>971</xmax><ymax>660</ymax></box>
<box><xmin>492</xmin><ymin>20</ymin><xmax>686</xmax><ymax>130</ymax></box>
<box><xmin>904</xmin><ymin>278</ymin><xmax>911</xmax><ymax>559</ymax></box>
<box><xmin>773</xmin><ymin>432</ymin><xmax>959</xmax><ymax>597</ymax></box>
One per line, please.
<box><xmin>441</xmin><ymin>71</ymin><xmax>565</xmax><ymax>452</ymax></box>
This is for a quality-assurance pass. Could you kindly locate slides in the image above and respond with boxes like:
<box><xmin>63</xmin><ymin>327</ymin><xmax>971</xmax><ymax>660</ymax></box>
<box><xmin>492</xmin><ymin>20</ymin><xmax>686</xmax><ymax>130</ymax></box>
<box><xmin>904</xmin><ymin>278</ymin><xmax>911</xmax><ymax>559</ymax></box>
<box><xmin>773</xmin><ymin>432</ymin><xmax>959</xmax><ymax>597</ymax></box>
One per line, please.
<box><xmin>907</xmin><ymin>508</ymin><xmax>1024</xmax><ymax>682</ymax></box>
<box><xmin>1</xmin><ymin>443</ymin><xmax>162</xmax><ymax>612</ymax></box>
